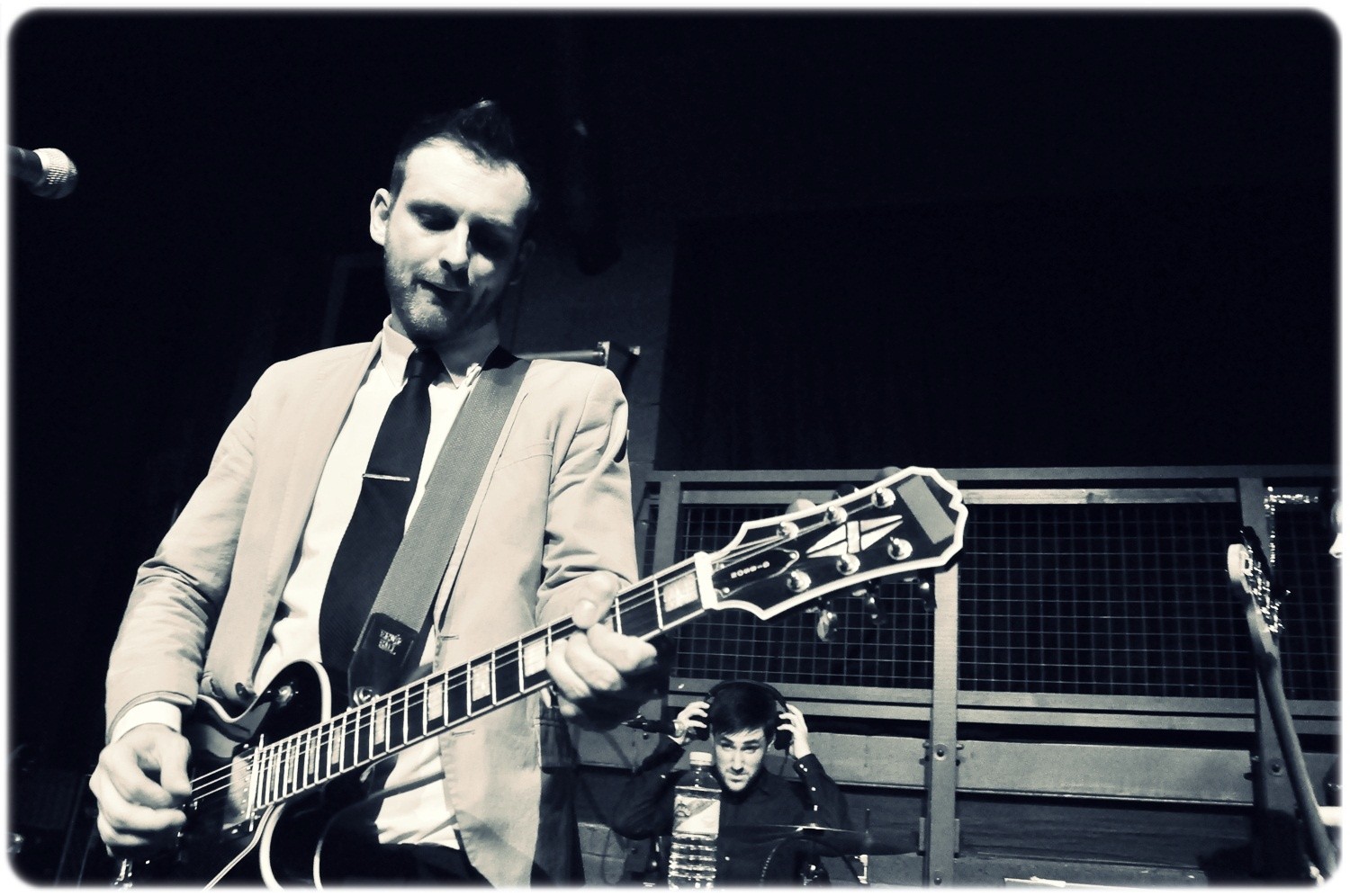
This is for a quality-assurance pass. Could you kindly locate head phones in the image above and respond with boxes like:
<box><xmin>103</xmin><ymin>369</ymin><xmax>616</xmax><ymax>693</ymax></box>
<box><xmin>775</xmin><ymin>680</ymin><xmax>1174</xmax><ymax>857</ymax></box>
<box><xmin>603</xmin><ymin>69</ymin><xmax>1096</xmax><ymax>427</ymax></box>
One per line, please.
<box><xmin>692</xmin><ymin>678</ymin><xmax>794</xmax><ymax>749</ymax></box>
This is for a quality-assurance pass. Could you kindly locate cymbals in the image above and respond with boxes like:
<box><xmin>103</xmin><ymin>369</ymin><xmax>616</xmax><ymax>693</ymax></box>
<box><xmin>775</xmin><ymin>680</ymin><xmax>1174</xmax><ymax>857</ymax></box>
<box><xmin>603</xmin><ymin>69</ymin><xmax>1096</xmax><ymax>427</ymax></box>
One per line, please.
<box><xmin>718</xmin><ymin>824</ymin><xmax>918</xmax><ymax>856</ymax></box>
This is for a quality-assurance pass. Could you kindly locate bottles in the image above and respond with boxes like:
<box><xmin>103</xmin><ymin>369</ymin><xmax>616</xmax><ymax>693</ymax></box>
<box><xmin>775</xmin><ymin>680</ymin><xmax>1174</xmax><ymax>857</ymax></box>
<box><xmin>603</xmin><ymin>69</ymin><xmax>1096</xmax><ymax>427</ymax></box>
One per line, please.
<box><xmin>667</xmin><ymin>751</ymin><xmax>722</xmax><ymax>889</ymax></box>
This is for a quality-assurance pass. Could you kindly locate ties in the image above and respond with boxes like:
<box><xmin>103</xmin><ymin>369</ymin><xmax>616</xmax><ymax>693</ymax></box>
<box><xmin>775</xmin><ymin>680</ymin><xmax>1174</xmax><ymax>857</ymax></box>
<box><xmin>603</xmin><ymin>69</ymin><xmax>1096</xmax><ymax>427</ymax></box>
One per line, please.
<box><xmin>318</xmin><ymin>343</ymin><xmax>446</xmax><ymax>720</ymax></box>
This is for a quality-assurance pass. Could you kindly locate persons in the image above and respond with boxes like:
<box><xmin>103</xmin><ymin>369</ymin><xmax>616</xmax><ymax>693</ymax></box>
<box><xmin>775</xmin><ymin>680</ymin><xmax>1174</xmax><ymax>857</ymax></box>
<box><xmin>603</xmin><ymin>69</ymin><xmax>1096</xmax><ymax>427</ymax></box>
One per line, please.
<box><xmin>1305</xmin><ymin>493</ymin><xmax>1344</xmax><ymax>885</ymax></box>
<box><xmin>88</xmin><ymin>109</ymin><xmax>666</xmax><ymax>887</ymax></box>
<box><xmin>611</xmin><ymin>680</ymin><xmax>856</xmax><ymax>886</ymax></box>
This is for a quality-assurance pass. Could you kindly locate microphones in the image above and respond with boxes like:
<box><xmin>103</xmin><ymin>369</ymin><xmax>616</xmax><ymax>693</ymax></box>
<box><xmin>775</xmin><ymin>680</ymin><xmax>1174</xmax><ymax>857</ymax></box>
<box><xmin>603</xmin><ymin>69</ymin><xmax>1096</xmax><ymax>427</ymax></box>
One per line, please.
<box><xmin>8</xmin><ymin>145</ymin><xmax>78</xmax><ymax>199</ymax></box>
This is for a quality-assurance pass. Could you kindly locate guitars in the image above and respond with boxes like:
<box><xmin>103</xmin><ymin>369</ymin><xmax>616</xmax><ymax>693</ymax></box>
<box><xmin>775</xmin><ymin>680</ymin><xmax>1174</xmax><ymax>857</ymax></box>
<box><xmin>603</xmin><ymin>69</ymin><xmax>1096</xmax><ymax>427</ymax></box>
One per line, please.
<box><xmin>75</xmin><ymin>465</ymin><xmax>967</xmax><ymax>889</ymax></box>
<box><xmin>1225</xmin><ymin>540</ymin><xmax>1339</xmax><ymax>880</ymax></box>
